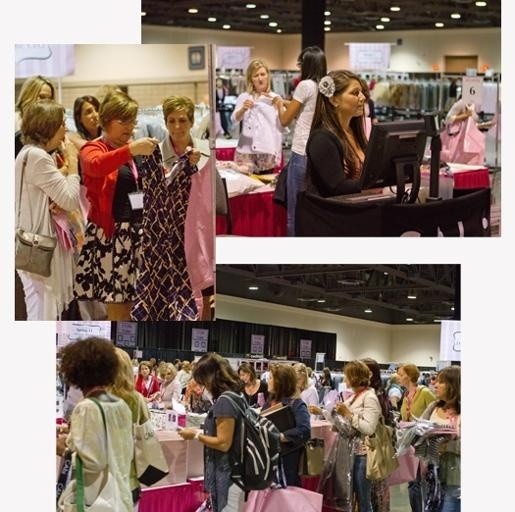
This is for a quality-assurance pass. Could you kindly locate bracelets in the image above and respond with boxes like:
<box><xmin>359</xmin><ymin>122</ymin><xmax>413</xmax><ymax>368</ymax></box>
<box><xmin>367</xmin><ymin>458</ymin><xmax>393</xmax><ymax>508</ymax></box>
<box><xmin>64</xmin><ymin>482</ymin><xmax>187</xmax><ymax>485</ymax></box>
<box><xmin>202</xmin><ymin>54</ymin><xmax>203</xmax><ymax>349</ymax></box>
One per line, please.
<box><xmin>481</xmin><ymin>123</ymin><xmax>485</xmax><ymax>128</ymax></box>
<box><xmin>275</xmin><ymin>99</ymin><xmax>283</xmax><ymax>105</ymax></box>
<box><xmin>475</xmin><ymin>122</ymin><xmax>479</xmax><ymax>129</ymax></box>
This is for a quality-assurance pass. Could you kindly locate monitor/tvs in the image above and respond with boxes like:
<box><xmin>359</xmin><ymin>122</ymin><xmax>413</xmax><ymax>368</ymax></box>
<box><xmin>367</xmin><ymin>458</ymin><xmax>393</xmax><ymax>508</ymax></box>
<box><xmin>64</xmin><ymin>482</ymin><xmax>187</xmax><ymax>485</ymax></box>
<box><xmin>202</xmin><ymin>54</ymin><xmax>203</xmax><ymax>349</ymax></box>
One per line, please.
<box><xmin>358</xmin><ymin>120</ymin><xmax>427</xmax><ymax>204</ymax></box>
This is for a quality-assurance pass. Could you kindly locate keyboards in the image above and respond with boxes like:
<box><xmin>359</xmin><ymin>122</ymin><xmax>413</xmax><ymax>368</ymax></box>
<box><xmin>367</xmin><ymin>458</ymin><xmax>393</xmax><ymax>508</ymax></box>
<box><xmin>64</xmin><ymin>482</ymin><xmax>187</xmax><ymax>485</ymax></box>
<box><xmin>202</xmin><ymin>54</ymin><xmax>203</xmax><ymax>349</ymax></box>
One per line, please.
<box><xmin>329</xmin><ymin>193</ymin><xmax>397</xmax><ymax>204</ymax></box>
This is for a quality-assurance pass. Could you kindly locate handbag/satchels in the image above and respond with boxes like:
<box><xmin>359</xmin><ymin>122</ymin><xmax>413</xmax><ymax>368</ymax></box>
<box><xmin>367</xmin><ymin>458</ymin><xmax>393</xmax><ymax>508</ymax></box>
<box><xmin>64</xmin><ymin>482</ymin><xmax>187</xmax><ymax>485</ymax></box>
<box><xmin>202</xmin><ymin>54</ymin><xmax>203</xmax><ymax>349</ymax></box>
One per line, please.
<box><xmin>16</xmin><ymin>231</ymin><xmax>57</xmax><ymax>276</ymax></box>
<box><xmin>364</xmin><ymin>419</ymin><xmax>401</xmax><ymax>482</ymax></box>
<box><xmin>439</xmin><ymin>117</ymin><xmax>484</xmax><ymax>164</ymax></box>
<box><xmin>134</xmin><ymin>420</ymin><xmax>169</xmax><ymax>485</ymax></box>
<box><xmin>300</xmin><ymin>439</ymin><xmax>324</xmax><ymax>476</ymax></box>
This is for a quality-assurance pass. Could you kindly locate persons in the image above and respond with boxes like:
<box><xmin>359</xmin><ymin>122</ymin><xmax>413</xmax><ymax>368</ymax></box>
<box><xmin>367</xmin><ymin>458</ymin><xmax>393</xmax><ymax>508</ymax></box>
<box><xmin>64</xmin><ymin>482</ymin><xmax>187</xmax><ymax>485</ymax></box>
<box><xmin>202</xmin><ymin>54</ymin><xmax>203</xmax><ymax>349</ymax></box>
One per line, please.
<box><xmin>215</xmin><ymin>68</ymin><xmax>222</xmax><ymax>76</ymax></box>
<box><xmin>214</xmin><ymin>78</ymin><xmax>233</xmax><ymax>140</ymax></box>
<box><xmin>360</xmin><ymin>79</ymin><xmax>378</xmax><ymax>126</ymax></box>
<box><xmin>16</xmin><ymin>74</ymin><xmax>216</xmax><ymax>321</ymax></box>
<box><xmin>290</xmin><ymin>72</ymin><xmax>303</xmax><ymax>99</ymax></box>
<box><xmin>425</xmin><ymin>92</ymin><xmax>497</xmax><ymax>166</ymax></box>
<box><xmin>448</xmin><ymin>77</ymin><xmax>457</xmax><ymax>109</ymax></box>
<box><xmin>229</xmin><ymin>59</ymin><xmax>291</xmax><ymax>176</ymax></box>
<box><xmin>304</xmin><ymin>68</ymin><xmax>385</xmax><ymax>198</ymax></box>
<box><xmin>56</xmin><ymin>336</ymin><xmax>460</xmax><ymax>512</ymax></box>
<box><xmin>271</xmin><ymin>45</ymin><xmax>328</xmax><ymax>235</ymax></box>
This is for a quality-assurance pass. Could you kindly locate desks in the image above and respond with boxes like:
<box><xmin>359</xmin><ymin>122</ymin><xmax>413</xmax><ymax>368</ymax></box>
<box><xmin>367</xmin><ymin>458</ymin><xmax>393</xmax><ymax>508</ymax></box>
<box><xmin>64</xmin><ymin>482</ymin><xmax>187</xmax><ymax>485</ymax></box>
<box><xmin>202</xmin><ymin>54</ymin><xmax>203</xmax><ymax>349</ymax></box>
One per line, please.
<box><xmin>420</xmin><ymin>162</ymin><xmax>491</xmax><ymax>189</ymax></box>
<box><xmin>222</xmin><ymin>172</ymin><xmax>286</xmax><ymax>237</ymax></box>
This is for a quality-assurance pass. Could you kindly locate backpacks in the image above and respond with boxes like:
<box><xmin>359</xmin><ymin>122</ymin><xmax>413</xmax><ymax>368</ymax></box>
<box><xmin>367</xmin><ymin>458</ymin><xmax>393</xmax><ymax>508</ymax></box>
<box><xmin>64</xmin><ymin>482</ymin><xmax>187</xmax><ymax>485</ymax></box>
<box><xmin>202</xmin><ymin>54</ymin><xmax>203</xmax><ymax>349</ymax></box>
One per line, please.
<box><xmin>216</xmin><ymin>392</ymin><xmax>281</xmax><ymax>490</ymax></box>
